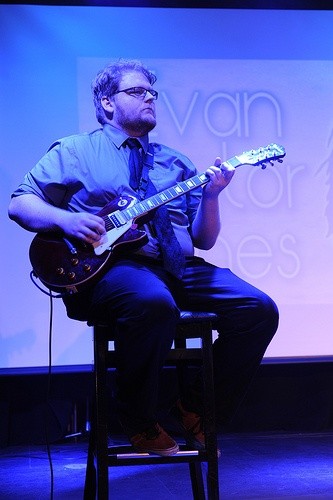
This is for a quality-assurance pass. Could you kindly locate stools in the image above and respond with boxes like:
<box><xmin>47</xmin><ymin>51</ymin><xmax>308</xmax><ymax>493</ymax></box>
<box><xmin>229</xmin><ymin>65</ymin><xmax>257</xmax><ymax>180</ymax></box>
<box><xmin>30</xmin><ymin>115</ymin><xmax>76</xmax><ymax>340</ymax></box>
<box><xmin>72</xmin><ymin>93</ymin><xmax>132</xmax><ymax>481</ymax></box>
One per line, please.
<box><xmin>83</xmin><ymin>313</ymin><xmax>220</xmax><ymax>500</ymax></box>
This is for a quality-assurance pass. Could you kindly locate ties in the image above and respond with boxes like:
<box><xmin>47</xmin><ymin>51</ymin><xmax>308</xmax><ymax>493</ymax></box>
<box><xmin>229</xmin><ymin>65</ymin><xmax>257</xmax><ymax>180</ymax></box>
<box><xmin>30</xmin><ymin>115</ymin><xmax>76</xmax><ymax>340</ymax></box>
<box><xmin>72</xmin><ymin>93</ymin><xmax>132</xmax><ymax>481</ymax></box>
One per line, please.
<box><xmin>125</xmin><ymin>138</ymin><xmax>185</xmax><ymax>275</ymax></box>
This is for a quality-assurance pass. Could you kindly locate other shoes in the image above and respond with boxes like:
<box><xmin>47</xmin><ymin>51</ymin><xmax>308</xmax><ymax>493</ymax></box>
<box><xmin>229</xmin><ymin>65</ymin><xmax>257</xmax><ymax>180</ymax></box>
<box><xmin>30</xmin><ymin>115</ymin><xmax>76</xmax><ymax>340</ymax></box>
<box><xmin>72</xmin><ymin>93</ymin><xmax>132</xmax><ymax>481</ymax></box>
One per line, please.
<box><xmin>128</xmin><ymin>423</ymin><xmax>179</xmax><ymax>456</ymax></box>
<box><xmin>177</xmin><ymin>399</ymin><xmax>220</xmax><ymax>458</ymax></box>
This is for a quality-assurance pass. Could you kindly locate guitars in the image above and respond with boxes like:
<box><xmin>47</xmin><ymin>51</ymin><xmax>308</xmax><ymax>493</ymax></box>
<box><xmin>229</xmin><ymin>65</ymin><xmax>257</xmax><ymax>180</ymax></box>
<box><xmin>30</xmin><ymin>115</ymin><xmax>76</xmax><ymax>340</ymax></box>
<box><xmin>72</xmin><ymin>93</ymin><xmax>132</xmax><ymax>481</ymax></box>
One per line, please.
<box><xmin>28</xmin><ymin>140</ymin><xmax>289</xmax><ymax>293</ymax></box>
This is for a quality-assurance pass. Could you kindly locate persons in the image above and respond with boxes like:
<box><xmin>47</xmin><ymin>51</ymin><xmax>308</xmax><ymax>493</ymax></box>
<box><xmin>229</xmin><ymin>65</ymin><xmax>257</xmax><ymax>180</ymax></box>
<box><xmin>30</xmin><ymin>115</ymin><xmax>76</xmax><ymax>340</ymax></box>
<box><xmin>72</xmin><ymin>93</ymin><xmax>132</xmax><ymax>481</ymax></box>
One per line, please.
<box><xmin>8</xmin><ymin>58</ymin><xmax>279</xmax><ymax>457</ymax></box>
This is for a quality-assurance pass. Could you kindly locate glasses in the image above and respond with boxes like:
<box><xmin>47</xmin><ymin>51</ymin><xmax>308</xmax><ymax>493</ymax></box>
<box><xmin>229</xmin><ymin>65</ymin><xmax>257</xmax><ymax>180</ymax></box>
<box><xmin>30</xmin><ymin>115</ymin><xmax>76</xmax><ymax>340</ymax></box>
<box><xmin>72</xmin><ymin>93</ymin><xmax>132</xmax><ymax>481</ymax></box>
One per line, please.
<box><xmin>107</xmin><ymin>87</ymin><xmax>158</xmax><ymax>100</ymax></box>
